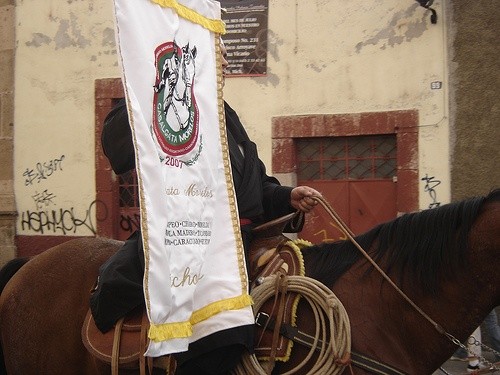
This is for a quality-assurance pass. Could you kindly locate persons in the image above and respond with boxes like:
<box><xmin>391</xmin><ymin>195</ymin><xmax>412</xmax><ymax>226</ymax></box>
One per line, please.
<box><xmin>99</xmin><ymin>31</ymin><xmax>323</xmax><ymax>375</ymax></box>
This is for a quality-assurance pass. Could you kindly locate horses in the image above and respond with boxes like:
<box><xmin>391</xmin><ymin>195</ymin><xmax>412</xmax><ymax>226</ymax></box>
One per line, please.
<box><xmin>0</xmin><ymin>186</ymin><xmax>500</xmax><ymax>375</ymax></box>
